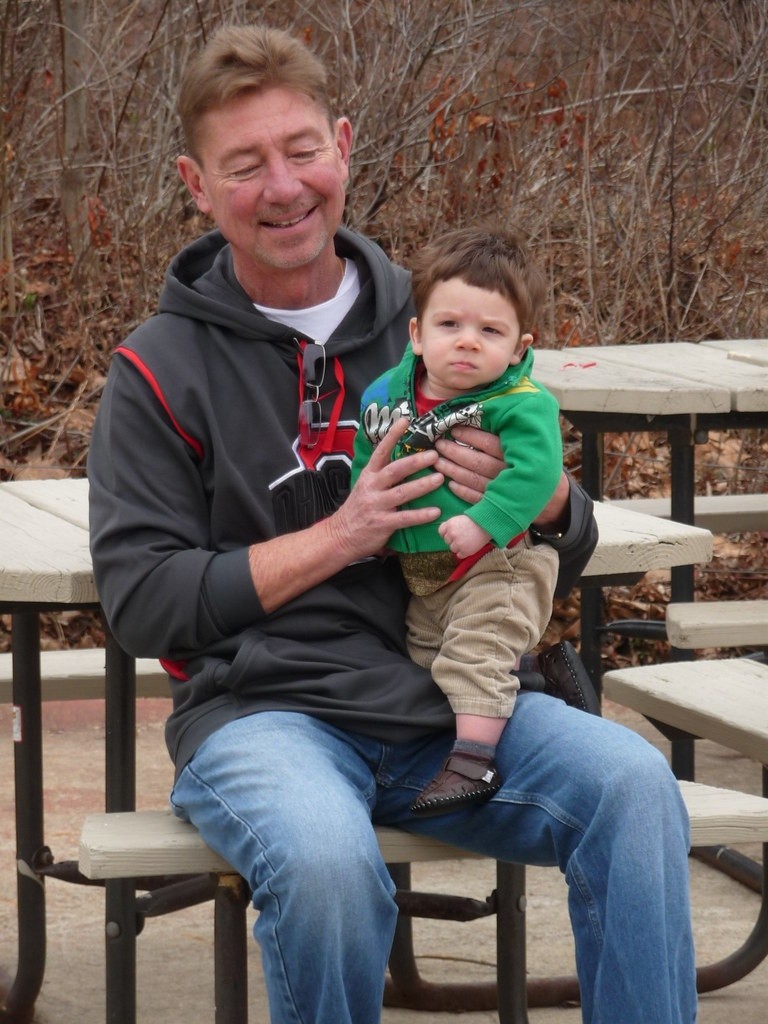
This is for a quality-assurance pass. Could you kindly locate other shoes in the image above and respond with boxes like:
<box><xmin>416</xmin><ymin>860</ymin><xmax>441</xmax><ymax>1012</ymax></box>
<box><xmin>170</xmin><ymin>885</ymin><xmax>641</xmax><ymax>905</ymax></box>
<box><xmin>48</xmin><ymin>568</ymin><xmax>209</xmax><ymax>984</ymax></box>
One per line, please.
<box><xmin>539</xmin><ymin>640</ymin><xmax>601</xmax><ymax>717</ymax></box>
<box><xmin>410</xmin><ymin>756</ymin><xmax>502</xmax><ymax>817</ymax></box>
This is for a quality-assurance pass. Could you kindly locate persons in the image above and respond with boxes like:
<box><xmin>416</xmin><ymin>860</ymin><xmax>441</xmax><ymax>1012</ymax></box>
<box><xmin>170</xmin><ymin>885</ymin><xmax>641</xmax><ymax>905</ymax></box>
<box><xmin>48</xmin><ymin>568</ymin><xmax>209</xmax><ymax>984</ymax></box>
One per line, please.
<box><xmin>87</xmin><ymin>24</ymin><xmax>698</xmax><ymax>1024</ymax></box>
<box><xmin>351</xmin><ymin>228</ymin><xmax>560</xmax><ymax>813</ymax></box>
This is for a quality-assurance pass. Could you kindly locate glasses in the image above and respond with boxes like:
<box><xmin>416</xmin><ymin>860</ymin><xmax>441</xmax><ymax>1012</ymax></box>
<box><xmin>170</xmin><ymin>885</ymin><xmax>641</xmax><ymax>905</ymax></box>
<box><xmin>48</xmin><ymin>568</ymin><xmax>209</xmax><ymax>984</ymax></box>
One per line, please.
<box><xmin>289</xmin><ymin>335</ymin><xmax>325</xmax><ymax>449</ymax></box>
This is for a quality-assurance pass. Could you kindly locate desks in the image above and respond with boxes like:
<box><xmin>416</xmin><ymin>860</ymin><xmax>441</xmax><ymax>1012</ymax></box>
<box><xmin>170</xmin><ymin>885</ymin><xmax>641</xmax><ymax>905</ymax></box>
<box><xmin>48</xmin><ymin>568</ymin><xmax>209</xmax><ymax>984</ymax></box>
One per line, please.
<box><xmin>530</xmin><ymin>339</ymin><xmax>768</xmax><ymax>780</ymax></box>
<box><xmin>0</xmin><ymin>478</ymin><xmax>712</xmax><ymax>1023</ymax></box>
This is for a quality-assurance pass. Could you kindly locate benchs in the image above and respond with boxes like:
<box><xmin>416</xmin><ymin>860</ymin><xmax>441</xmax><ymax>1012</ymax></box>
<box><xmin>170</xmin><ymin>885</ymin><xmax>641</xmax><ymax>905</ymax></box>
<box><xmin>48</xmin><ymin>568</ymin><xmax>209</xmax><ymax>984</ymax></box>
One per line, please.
<box><xmin>77</xmin><ymin>490</ymin><xmax>767</xmax><ymax>1021</ymax></box>
